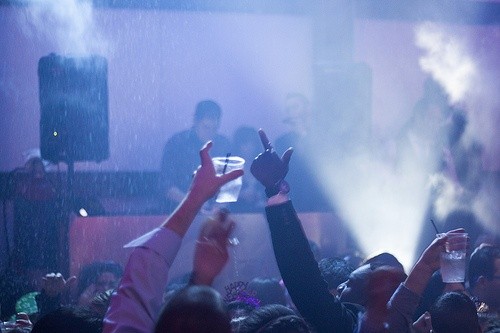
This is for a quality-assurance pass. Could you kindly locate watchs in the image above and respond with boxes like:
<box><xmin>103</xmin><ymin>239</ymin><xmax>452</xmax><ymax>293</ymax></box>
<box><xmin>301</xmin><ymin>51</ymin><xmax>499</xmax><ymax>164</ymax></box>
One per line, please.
<box><xmin>265</xmin><ymin>177</ymin><xmax>291</xmax><ymax>197</ymax></box>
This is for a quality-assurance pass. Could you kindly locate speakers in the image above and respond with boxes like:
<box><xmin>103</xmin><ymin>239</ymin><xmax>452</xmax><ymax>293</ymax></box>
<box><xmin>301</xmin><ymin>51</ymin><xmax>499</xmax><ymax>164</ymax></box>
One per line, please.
<box><xmin>38</xmin><ymin>52</ymin><xmax>110</xmax><ymax>165</ymax></box>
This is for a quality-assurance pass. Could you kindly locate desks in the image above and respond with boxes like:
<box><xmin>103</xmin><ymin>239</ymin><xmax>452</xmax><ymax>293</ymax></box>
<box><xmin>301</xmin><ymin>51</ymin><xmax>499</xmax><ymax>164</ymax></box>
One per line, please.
<box><xmin>71</xmin><ymin>212</ymin><xmax>347</xmax><ymax>291</ymax></box>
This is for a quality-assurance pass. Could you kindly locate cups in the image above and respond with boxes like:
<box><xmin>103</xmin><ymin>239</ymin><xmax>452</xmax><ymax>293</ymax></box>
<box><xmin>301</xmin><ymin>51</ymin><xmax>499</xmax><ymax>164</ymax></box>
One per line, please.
<box><xmin>435</xmin><ymin>232</ymin><xmax>468</xmax><ymax>283</ymax></box>
<box><xmin>211</xmin><ymin>156</ymin><xmax>245</xmax><ymax>203</ymax></box>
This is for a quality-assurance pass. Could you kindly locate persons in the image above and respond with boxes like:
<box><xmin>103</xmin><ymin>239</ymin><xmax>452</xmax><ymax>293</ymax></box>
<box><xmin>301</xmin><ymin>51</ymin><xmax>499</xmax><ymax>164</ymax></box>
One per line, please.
<box><xmin>402</xmin><ymin>96</ymin><xmax>468</xmax><ymax>172</ymax></box>
<box><xmin>102</xmin><ymin>139</ymin><xmax>243</xmax><ymax>333</ymax></box>
<box><xmin>224</xmin><ymin>276</ymin><xmax>309</xmax><ymax>333</ymax></box>
<box><xmin>318</xmin><ymin>257</ymin><xmax>354</xmax><ymax>297</ymax></box>
<box><xmin>160</xmin><ymin>99</ymin><xmax>266</xmax><ymax>215</ymax></box>
<box><xmin>274</xmin><ymin>92</ymin><xmax>334</xmax><ymax>213</ymax></box>
<box><xmin>0</xmin><ymin>261</ymin><xmax>123</xmax><ymax>333</ymax></box>
<box><xmin>250</xmin><ymin>128</ymin><xmax>408</xmax><ymax>333</ymax></box>
<box><xmin>357</xmin><ymin>227</ymin><xmax>500</xmax><ymax>333</ymax></box>
